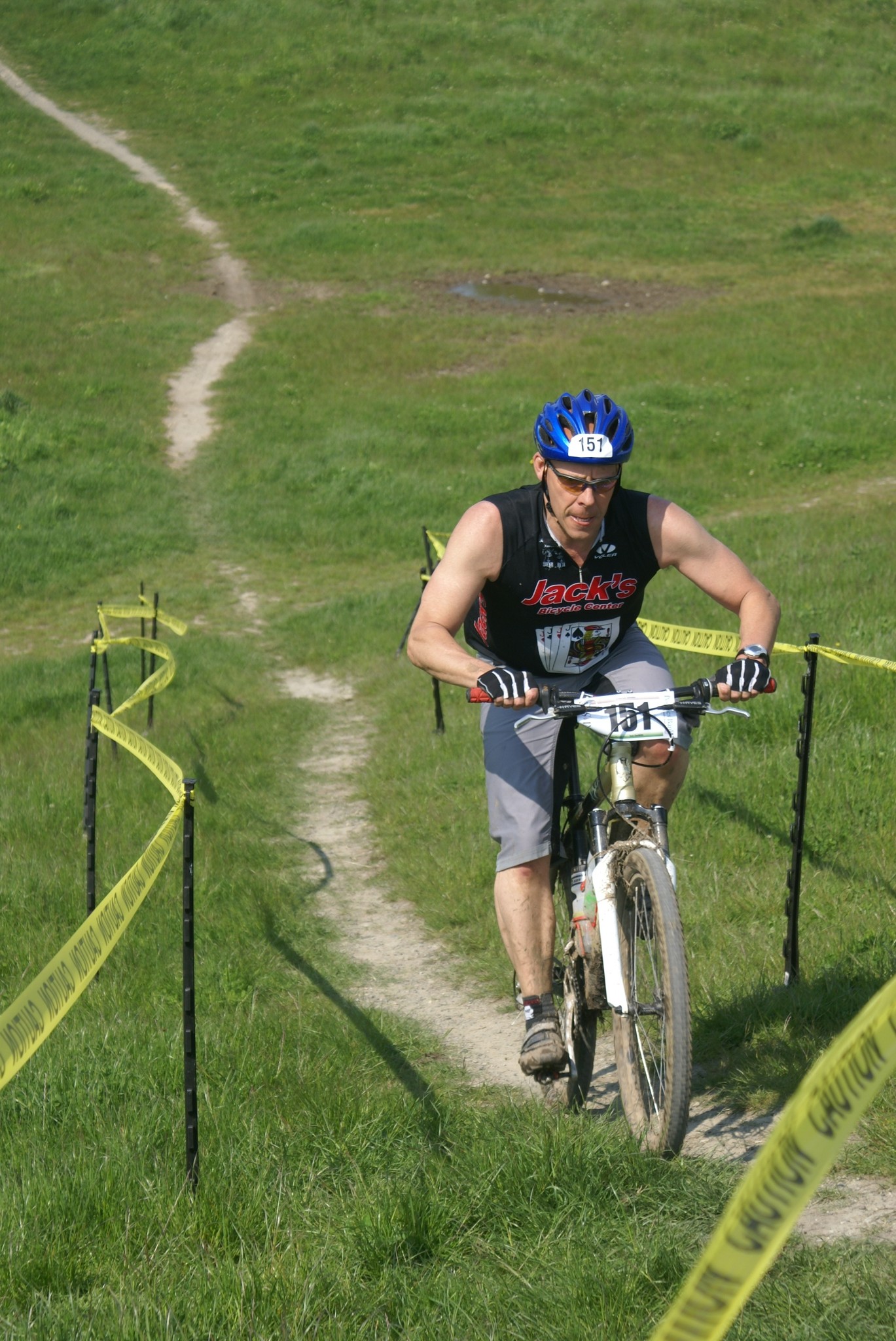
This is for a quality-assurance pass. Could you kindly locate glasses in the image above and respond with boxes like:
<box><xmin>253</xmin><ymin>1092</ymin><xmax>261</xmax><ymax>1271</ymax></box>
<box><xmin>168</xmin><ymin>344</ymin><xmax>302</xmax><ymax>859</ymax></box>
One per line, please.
<box><xmin>544</xmin><ymin>457</ymin><xmax>623</xmax><ymax>494</ymax></box>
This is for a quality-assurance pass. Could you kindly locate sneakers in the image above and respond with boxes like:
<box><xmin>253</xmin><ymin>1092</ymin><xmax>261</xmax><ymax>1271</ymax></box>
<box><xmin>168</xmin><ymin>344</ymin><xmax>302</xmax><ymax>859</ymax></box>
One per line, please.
<box><xmin>635</xmin><ymin>891</ymin><xmax>653</xmax><ymax>939</ymax></box>
<box><xmin>515</xmin><ymin>992</ymin><xmax>567</xmax><ymax>1077</ymax></box>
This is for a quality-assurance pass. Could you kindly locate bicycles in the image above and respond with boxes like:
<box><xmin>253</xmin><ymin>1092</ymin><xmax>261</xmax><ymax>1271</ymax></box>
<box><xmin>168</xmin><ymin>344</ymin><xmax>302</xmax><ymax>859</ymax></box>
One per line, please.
<box><xmin>466</xmin><ymin>675</ymin><xmax>778</xmax><ymax>1160</ymax></box>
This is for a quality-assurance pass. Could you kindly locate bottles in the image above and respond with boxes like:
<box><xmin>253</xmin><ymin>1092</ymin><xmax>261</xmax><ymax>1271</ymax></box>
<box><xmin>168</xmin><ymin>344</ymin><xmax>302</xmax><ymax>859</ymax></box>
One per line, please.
<box><xmin>570</xmin><ymin>859</ymin><xmax>595</xmax><ymax>956</ymax></box>
<box><xmin>584</xmin><ymin>847</ymin><xmax>597</xmax><ymax>931</ymax></box>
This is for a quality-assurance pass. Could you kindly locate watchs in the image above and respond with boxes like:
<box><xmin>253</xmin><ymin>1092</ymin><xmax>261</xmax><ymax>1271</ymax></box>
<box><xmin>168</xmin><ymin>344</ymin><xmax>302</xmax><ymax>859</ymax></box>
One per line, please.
<box><xmin>735</xmin><ymin>644</ymin><xmax>770</xmax><ymax>667</ymax></box>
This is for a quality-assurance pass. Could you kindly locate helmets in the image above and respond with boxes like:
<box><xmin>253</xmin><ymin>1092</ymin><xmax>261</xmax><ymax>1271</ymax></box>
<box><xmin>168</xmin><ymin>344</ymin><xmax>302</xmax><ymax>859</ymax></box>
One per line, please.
<box><xmin>531</xmin><ymin>388</ymin><xmax>636</xmax><ymax>465</ymax></box>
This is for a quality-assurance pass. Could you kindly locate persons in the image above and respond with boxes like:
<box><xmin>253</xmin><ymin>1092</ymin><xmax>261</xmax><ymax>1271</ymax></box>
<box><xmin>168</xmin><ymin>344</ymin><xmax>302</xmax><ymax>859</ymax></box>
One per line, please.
<box><xmin>405</xmin><ymin>390</ymin><xmax>782</xmax><ymax>1073</ymax></box>
